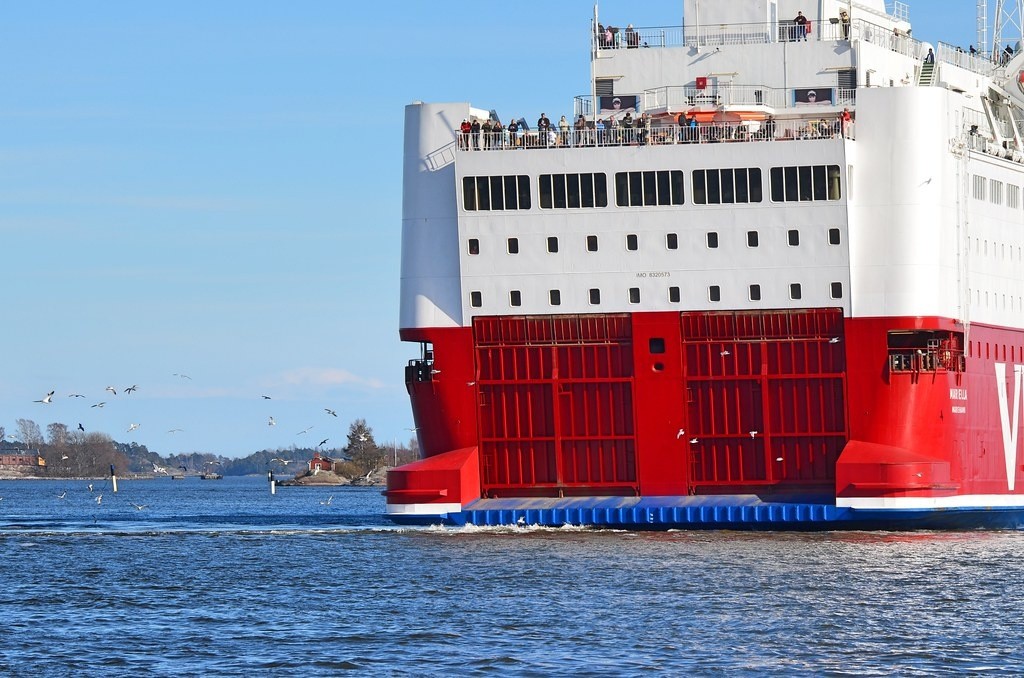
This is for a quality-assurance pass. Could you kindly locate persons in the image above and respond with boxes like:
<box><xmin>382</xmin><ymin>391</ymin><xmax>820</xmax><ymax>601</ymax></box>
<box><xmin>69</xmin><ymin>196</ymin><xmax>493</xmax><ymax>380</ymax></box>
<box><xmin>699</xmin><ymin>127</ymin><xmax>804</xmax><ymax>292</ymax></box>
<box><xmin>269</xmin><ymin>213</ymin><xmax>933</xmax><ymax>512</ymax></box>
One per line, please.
<box><xmin>969</xmin><ymin>45</ymin><xmax>976</xmax><ymax>57</ymax></box>
<box><xmin>664</xmin><ymin>112</ymin><xmax>699</xmax><ymax>143</ymax></box>
<box><xmin>907</xmin><ymin>29</ymin><xmax>912</xmax><ymax>35</ymax></box>
<box><xmin>706</xmin><ymin>121</ymin><xmax>745</xmax><ymax>140</ymax></box>
<box><xmin>841</xmin><ymin>12</ymin><xmax>849</xmax><ymax>39</ymax></box>
<box><xmin>925</xmin><ymin>48</ymin><xmax>934</xmax><ymax>64</ymax></box>
<box><xmin>969</xmin><ymin>125</ymin><xmax>982</xmax><ymax>137</ymax></box>
<box><xmin>798</xmin><ymin>108</ymin><xmax>851</xmax><ymax>139</ymax></box>
<box><xmin>601</xmin><ymin>98</ymin><xmax>635</xmax><ymax>112</ymax></box>
<box><xmin>575</xmin><ymin>113</ymin><xmax>647</xmax><ymax>147</ymax></box>
<box><xmin>594</xmin><ymin>23</ymin><xmax>619</xmax><ymax>49</ymax></box>
<box><xmin>956</xmin><ymin>47</ymin><xmax>962</xmax><ymax>53</ymax></box>
<box><xmin>1004</xmin><ymin>45</ymin><xmax>1013</xmax><ymax>63</ymax></box>
<box><xmin>749</xmin><ymin>116</ymin><xmax>776</xmax><ymax>141</ymax></box>
<box><xmin>796</xmin><ymin>91</ymin><xmax>831</xmax><ymax>104</ymax></box>
<box><xmin>794</xmin><ymin>11</ymin><xmax>807</xmax><ymax>42</ymax></box>
<box><xmin>462</xmin><ymin>113</ymin><xmax>570</xmax><ymax>151</ymax></box>
<box><xmin>625</xmin><ymin>24</ymin><xmax>638</xmax><ymax>49</ymax></box>
<box><xmin>862</xmin><ymin>26</ymin><xmax>872</xmax><ymax>42</ymax></box>
<box><xmin>892</xmin><ymin>28</ymin><xmax>898</xmax><ymax>52</ymax></box>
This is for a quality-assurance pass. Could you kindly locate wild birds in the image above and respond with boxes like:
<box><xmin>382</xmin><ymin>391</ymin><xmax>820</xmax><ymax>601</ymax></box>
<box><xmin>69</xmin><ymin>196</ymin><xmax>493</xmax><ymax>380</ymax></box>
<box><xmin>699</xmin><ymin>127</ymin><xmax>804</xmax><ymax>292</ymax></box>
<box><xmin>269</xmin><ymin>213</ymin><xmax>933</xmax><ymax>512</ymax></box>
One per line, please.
<box><xmin>172</xmin><ymin>373</ymin><xmax>191</xmax><ymax>380</ymax></box>
<box><xmin>77</xmin><ymin>423</ymin><xmax>85</xmax><ymax>431</ymax></box>
<box><xmin>124</xmin><ymin>385</ymin><xmax>137</xmax><ymax>394</ymax></box>
<box><xmin>61</xmin><ymin>455</ymin><xmax>68</xmax><ymax>460</ymax></box>
<box><xmin>86</xmin><ymin>484</ymin><xmax>93</xmax><ymax>491</ymax></box>
<box><xmin>261</xmin><ymin>394</ymin><xmax>422</xmax><ymax>506</ymax></box>
<box><xmin>177</xmin><ymin>465</ymin><xmax>187</xmax><ymax>472</ymax></box>
<box><xmin>91</xmin><ymin>402</ymin><xmax>106</xmax><ymax>408</ymax></box>
<box><xmin>127</xmin><ymin>423</ymin><xmax>140</xmax><ymax>432</ymax></box>
<box><xmin>32</xmin><ymin>391</ymin><xmax>55</xmax><ymax>403</ymax></box>
<box><xmin>106</xmin><ymin>387</ymin><xmax>117</xmax><ymax>395</ymax></box>
<box><xmin>152</xmin><ymin>463</ymin><xmax>168</xmax><ymax>476</ymax></box>
<box><xmin>96</xmin><ymin>494</ymin><xmax>102</xmax><ymax>503</ymax></box>
<box><xmin>129</xmin><ymin>502</ymin><xmax>148</xmax><ymax>510</ymax></box>
<box><xmin>68</xmin><ymin>393</ymin><xmax>86</xmax><ymax>399</ymax></box>
<box><xmin>6</xmin><ymin>434</ymin><xmax>17</xmax><ymax>439</ymax></box>
<box><xmin>166</xmin><ymin>428</ymin><xmax>182</xmax><ymax>433</ymax></box>
<box><xmin>202</xmin><ymin>461</ymin><xmax>221</xmax><ymax>466</ymax></box>
<box><xmin>56</xmin><ymin>490</ymin><xmax>67</xmax><ymax>499</ymax></box>
<box><xmin>674</xmin><ymin>334</ymin><xmax>840</xmax><ymax>449</ymax></box>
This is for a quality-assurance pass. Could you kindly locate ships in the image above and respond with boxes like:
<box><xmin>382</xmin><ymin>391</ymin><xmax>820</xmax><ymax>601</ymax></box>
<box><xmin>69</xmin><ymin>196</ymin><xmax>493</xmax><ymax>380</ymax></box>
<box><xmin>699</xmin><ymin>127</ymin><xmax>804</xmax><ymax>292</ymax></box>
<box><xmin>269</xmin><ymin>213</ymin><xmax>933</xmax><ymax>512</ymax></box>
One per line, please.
<box><xmin>382</xmin><ymin>0</ymin><xmax>1023</xmax><ymax>532</ymax></box>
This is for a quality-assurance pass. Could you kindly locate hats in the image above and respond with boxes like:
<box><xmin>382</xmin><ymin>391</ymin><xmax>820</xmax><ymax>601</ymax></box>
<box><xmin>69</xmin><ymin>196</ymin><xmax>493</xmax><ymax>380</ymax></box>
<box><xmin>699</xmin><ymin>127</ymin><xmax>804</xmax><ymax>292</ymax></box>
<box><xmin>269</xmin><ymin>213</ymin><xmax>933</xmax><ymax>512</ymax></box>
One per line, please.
<box><xmin>612</xmin><ymin>98</ymin><xmax>621</xmax><ymax>104</ymax></box>
<box><xmin>463</xmin><ymin>118</ymin><xmax>467</xmax><ymax>122</ymax></box>
<box><xmin>628</xmin><ymin>24</ymin><xmax>633</xmax><ymax>27</ymax></box>
<box><xmin>842</xmin><ymin>12</ymin><xmax>846</xmax><ymax>14</ymax></box>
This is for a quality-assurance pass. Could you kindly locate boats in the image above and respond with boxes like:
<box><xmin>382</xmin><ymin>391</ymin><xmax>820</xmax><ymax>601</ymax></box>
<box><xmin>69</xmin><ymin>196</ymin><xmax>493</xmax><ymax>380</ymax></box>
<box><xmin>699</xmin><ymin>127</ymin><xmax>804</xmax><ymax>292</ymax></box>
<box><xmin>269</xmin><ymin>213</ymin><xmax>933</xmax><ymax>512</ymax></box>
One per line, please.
<box><xmin>201</xmin><ymin>471</ymin><xmax>224</xmax><ymax>479</ymax></box>
<box><xmin>171</xmin><ymin>474</ymin><xmax>186</xmax><ymax>480</ymax></box>
